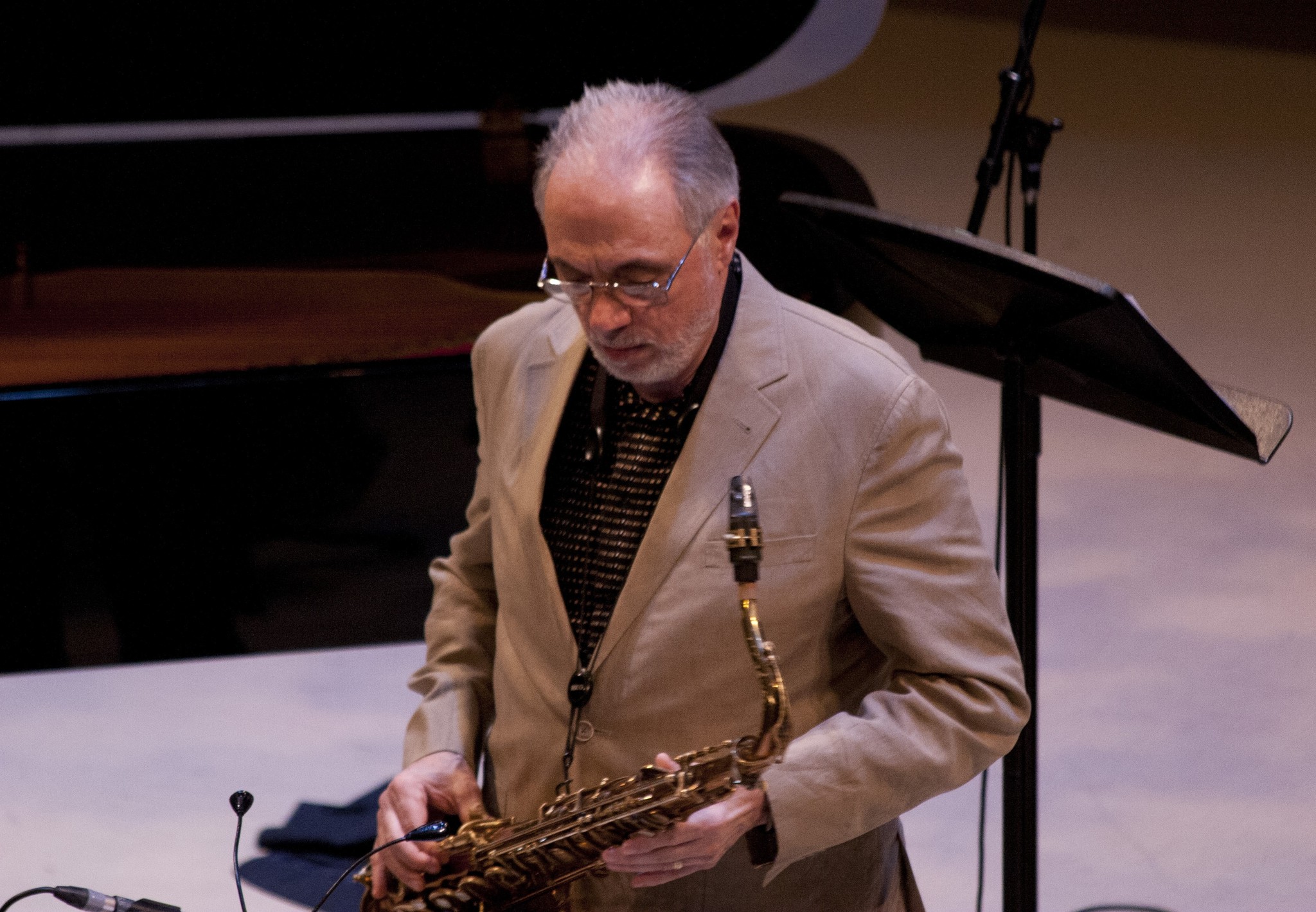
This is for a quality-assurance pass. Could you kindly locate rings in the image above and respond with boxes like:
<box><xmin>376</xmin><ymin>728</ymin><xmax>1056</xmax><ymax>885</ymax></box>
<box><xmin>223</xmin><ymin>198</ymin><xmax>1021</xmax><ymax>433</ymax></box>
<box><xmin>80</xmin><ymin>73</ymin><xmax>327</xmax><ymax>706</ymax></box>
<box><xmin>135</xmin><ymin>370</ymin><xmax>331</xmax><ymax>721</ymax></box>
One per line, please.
<box><xmin>673</xmin><ymin>860</ymin><xmax>683</xmax><ymax>871</ymax></box>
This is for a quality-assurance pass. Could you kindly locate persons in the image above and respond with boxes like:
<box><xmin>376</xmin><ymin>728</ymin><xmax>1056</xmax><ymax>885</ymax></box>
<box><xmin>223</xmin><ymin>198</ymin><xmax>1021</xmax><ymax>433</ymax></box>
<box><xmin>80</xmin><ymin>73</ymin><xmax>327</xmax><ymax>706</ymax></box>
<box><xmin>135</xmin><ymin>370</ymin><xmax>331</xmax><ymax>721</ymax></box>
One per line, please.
<box><xmin>368</xmin><ymin>77</ymin><xmax>1032</xmax><ymax>912</ymax></box>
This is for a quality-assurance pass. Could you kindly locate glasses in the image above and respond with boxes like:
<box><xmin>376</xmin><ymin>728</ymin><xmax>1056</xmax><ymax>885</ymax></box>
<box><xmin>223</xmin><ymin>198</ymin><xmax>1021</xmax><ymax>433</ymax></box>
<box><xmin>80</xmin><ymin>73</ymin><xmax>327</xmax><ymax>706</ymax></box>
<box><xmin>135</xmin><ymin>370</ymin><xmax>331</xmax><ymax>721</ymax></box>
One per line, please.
<box><xmin>537</xmin><ymin>206</ymin><xmax>724</xmax><ymax>307</ymax></box>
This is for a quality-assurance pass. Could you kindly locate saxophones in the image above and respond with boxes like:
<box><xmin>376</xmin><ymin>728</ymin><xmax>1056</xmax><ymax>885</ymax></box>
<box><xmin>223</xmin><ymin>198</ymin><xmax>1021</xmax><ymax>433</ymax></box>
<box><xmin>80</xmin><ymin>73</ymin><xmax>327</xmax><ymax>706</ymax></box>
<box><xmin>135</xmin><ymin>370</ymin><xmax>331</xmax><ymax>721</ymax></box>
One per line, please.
<box><xmin>357</xmin><ymin>476</ymin><xmax>790</xmax><ymax>912</ymax></box>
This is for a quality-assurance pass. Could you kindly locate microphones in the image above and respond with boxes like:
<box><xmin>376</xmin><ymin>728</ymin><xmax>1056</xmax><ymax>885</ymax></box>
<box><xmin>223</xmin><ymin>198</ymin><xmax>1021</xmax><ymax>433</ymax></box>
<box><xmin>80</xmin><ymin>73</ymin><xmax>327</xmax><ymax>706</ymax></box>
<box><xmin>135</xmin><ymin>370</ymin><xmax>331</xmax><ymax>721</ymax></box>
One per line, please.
<box><xmin>52</xmin><ymin>885</ymin><xmax>182</xmax><ymax>912</ymax></box>
<box><xmin>311</xmin><ymin>819</ymin><xmax>449</xmax><ymax>912</ymax></box>
<box><xmin>228</xmin><ymin>790</ymin><xmax>255</xmax><ymax>912</ymax></box>
